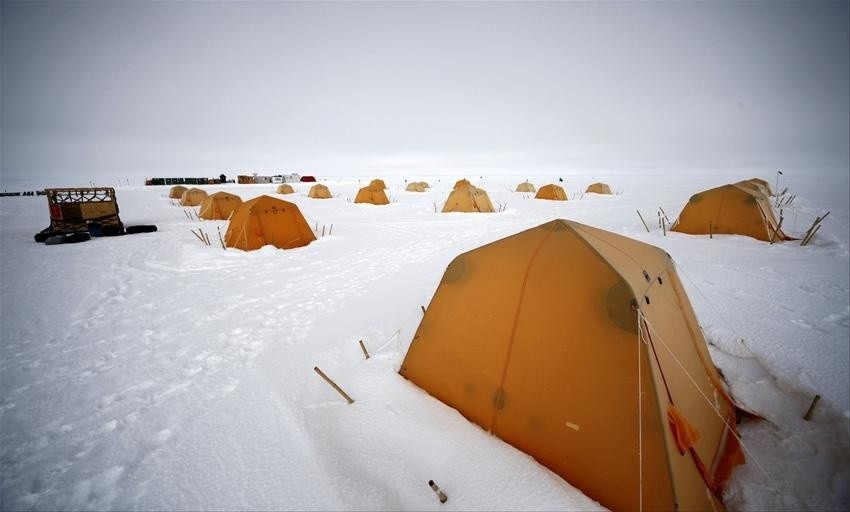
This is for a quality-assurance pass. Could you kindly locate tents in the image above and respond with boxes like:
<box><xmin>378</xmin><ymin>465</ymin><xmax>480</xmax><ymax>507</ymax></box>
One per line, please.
<box><xmin>535</xmin><ymin>184</ymin><xmax>567</xmax><ymax>200</ymax></box>
<box><xmin>168</xmin><ymin>178</ymin><xmax>431</xmax><ymax>253</ymax></box>
<box><xmin>399</xmin><ymin>217</ymin><xmax>764</xmax><ymax>511</ymax></box>
<box><xmin>586</xmin><ymin>182</ymin><xmax>611</xmax><ymax>195</ymax></box>
<box><xmin>669</xmin><ymin>177</ymin><xmax>787</xmax><ymax>244</ymax></box>
<box><xmin>442</xmin><ymin>179</ymin><xmax>495</xmax><ymax>213</ymax></box>
<box><xmin>515</xmin><ymin>181</ymin><xmax>535</xmax><ymax>192</ymax></box>
